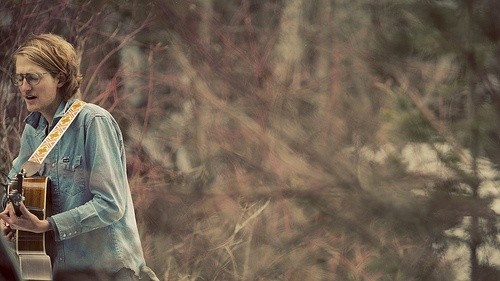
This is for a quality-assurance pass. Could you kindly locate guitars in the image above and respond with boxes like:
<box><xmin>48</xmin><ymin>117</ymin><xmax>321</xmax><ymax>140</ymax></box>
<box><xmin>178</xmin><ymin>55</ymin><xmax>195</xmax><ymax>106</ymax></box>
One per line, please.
<box><xmin>6</xmin><ymin>168</ymin><xmax>55</xmax><ymax>280</ymax></box>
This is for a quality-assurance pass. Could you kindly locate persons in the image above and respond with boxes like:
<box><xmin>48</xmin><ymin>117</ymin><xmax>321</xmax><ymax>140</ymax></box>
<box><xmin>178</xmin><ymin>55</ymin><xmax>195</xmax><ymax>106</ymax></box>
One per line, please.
<box><xmin>0</xmin><ymin>31</ymin><xmax>146</xmax><ymax>280</ymax></box>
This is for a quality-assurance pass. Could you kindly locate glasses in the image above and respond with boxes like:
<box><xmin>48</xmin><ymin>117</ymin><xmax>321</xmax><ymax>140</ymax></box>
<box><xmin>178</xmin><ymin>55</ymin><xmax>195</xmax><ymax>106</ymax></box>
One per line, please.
<box><xmin>10</xmin><ymin>72</ymin><xmax>49</xmax><ymax>86</ymax></box>
